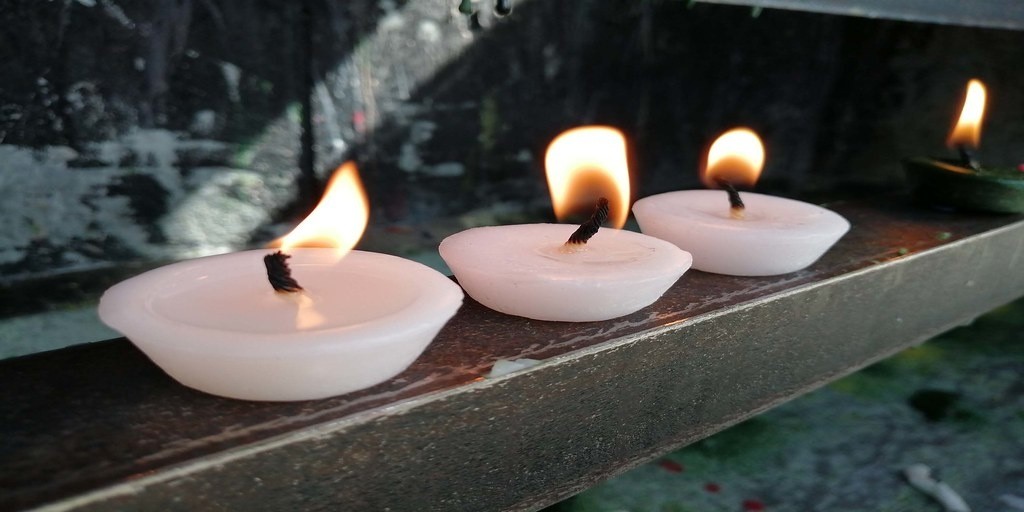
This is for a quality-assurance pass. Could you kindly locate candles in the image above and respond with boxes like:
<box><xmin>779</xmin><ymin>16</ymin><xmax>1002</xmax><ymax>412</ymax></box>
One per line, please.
<box><xmin>438</xmin><ymin>126</ymin><xmax>694</xmax><ymax>324</ymax></box>
<box><xmin>900</xmin><ymin>78</ymin><xmax>1024</xmax><ymax>214</ymax></box>
<box><xmin>99</xmin><ymin>162</ymin><xmax>465</xmax><ymax>403</ymax></box>
<box><xmin>630</xmin><ymin>126</ymin><xmax>851</xmax><ymax>277</ymax></box>
